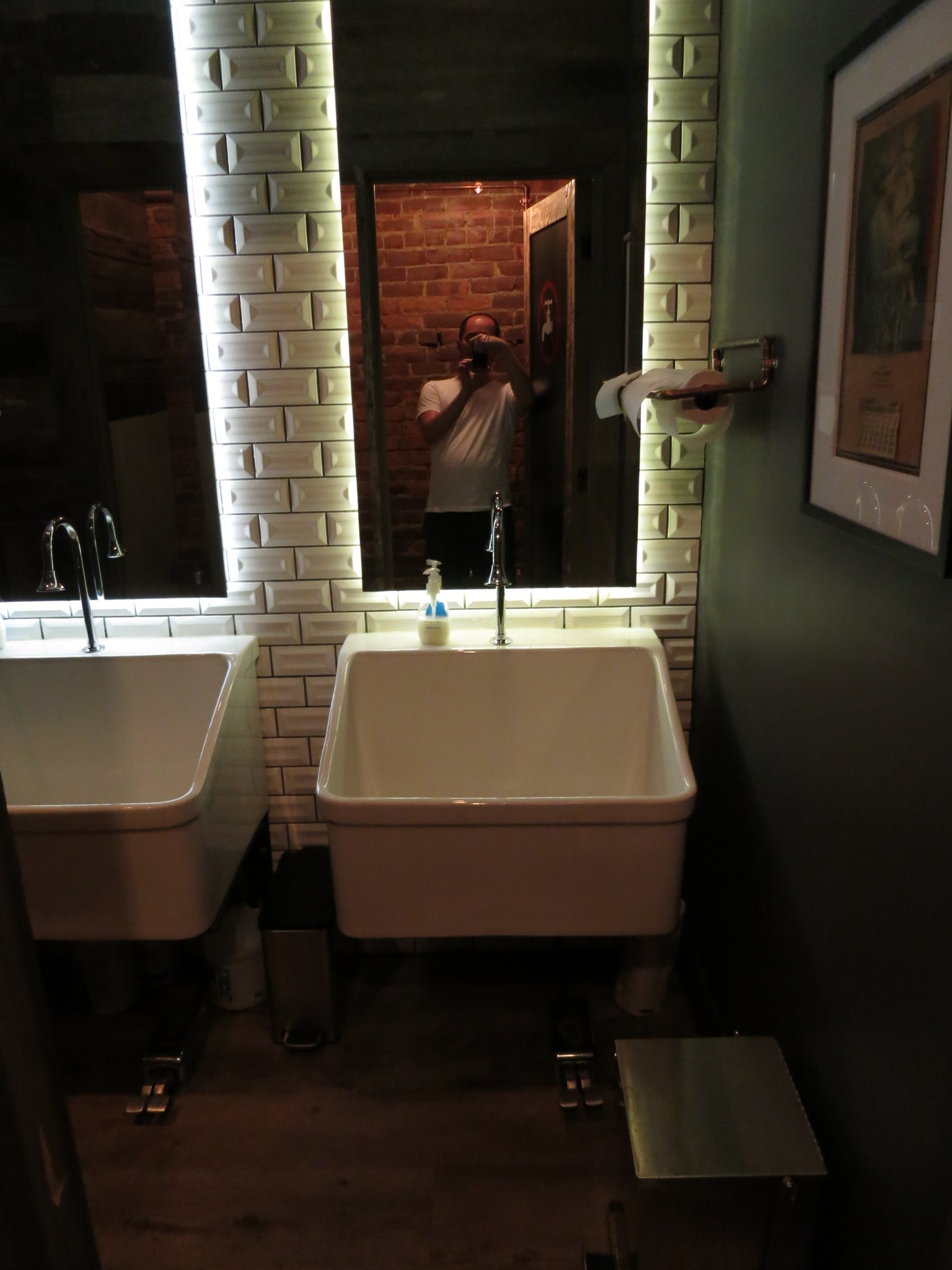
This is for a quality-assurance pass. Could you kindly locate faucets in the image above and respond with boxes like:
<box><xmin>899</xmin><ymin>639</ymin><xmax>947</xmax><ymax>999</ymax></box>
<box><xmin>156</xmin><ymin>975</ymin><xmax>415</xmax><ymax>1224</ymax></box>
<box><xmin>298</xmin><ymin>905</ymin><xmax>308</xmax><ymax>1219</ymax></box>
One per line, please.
<box><xmin>87</xmin><ymin>501</ymin><xmax>125</xmax><ymax>600</ymax></box>
<box><xmin>35</xmin><ymin>515</ymin><xmax>105</xmax><ymax>654</ymax></box>
<box><xmin>484</xmin><ymin>509</ymin><xmax>514</xmax><ymax>647</ymax></box>
<box><xmin>485</xmin><ymin>489</ymin><xmax>504</xmax><ymax>554</ymax></box>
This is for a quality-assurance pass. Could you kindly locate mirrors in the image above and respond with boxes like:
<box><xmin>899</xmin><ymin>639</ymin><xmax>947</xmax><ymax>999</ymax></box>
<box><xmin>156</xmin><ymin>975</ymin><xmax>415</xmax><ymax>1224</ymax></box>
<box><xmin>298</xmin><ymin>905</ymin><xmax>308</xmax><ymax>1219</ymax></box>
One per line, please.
<box><xmin>0</xmin><ymin>0</ymin><xmax>233</xmax><ymax>603</ymax></box>
<box><xmin>328</xmin><ymin>0</ymin><xmax>650</xmax><ymax>591</ymax></box>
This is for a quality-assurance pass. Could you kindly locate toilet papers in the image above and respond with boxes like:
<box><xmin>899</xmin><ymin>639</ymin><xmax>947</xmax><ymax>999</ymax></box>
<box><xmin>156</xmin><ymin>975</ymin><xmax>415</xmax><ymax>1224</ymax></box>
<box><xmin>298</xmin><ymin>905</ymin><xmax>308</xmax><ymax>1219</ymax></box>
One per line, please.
<box><xmin>621</xmin><ymin>365</ymin><xmax>734</xmax><ymax>450</ymax></box>
<box><xmin>595</xmin><ymin>370</ymin><xmax>642</xmax><ymax>421</ymax></box>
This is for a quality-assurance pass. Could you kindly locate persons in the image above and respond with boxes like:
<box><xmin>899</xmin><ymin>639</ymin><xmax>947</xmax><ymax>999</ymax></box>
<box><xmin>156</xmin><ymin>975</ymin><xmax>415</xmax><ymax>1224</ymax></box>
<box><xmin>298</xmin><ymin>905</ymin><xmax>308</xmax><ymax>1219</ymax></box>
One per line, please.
<box><xmin>415</xmin><ymin>313</ymin><xmax>535</xmax><ymax>590</ymax></box>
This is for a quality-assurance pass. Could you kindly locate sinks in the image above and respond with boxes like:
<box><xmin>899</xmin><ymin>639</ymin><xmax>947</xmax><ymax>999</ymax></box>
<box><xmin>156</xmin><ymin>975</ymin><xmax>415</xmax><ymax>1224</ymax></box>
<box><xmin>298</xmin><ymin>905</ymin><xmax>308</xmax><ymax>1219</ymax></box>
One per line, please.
<box><xmin>2</xmin><ymin>632</ymin><xmax>270</xmax><ymax>945</ymax></box>
<box><xmin>315</xmin><ymin>625</ymin><xmax>698</xmax><ymax>945</ymax></box>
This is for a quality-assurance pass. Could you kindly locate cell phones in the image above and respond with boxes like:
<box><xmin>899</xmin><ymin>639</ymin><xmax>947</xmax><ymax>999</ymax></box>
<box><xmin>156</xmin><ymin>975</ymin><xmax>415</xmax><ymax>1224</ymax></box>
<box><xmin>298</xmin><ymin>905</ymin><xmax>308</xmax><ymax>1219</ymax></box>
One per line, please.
<box><xmin>471</xmin><ymin>342</ymin><xmax>489</xmax><ymax>369</ymax></box>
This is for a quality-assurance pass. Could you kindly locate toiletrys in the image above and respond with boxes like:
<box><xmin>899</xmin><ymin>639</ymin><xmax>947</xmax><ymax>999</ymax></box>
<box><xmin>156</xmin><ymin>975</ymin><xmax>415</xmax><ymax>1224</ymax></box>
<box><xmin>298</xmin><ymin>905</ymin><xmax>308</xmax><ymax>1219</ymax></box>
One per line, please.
<box><xmin>417</xmin><ymin>557</ymin><xmax>449</xmax><ymax>646</ymax></box>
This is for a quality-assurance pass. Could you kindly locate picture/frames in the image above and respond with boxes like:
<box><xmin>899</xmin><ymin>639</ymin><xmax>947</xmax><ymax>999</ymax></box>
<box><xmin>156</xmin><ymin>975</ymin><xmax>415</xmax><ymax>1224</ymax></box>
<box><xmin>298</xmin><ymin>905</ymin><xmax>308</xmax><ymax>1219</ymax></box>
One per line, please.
<box><xmin>803</xmin><ymin>0</ymin><xmax>952</xmax><ymax>559</ymax></box>
